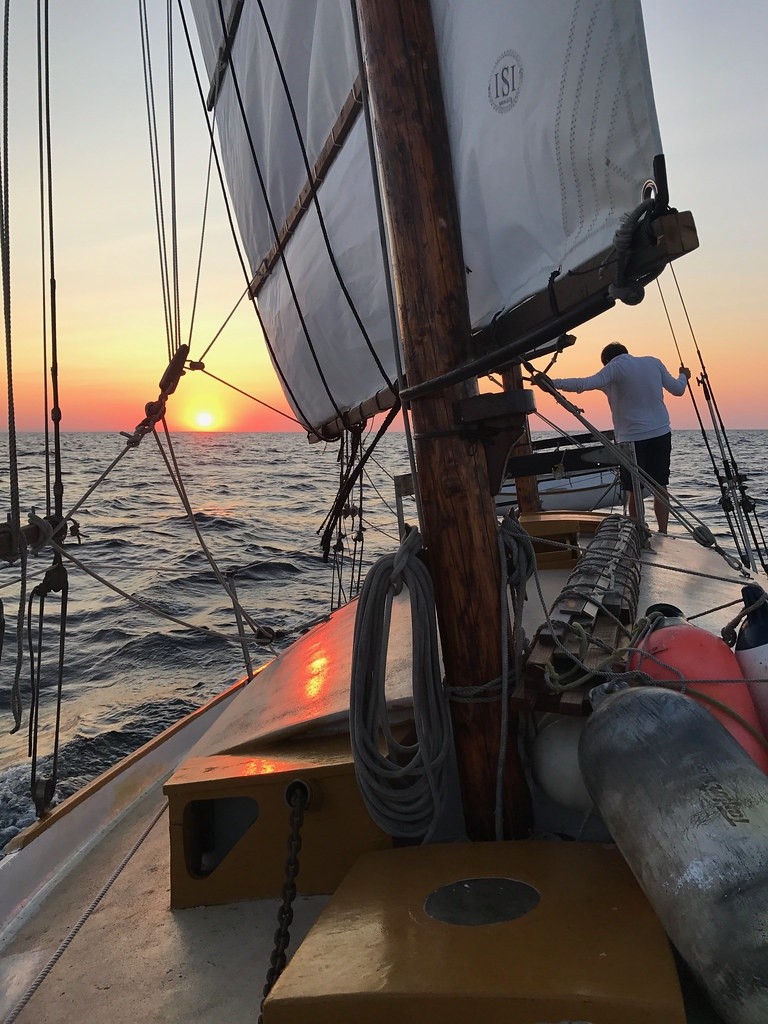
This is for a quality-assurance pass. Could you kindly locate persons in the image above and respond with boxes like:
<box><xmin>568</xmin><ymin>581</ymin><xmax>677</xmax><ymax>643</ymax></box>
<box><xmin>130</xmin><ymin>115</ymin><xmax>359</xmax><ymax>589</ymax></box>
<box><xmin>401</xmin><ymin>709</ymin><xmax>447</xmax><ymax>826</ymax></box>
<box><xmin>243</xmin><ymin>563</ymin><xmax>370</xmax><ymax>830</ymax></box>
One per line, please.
<box><xmin>530</xmin><ymin>342</ymin><xmax>690</xmax><ymax>537</ymax></box>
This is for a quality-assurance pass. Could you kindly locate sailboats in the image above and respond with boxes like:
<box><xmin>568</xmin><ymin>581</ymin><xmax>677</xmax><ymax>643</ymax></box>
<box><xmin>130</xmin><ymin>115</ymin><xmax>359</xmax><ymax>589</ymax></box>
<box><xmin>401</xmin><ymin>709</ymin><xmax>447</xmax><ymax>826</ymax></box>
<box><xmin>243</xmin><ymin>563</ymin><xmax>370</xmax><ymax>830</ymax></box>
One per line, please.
<box><xmin>1</xmin><ymin>2</ymin><xmax>768</xmax><ymax>1024</ymax></box>
